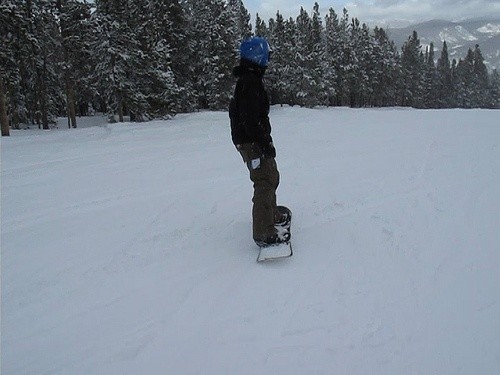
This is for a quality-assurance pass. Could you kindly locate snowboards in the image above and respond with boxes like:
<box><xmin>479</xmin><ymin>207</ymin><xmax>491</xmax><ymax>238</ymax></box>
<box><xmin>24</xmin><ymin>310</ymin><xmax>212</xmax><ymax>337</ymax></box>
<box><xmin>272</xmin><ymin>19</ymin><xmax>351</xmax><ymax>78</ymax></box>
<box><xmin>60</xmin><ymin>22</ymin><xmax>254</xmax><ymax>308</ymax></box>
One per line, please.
<box><xmin>256</xmin><ymin>206</ymin><xmax>293</xmax><ymax>263</ymax></box>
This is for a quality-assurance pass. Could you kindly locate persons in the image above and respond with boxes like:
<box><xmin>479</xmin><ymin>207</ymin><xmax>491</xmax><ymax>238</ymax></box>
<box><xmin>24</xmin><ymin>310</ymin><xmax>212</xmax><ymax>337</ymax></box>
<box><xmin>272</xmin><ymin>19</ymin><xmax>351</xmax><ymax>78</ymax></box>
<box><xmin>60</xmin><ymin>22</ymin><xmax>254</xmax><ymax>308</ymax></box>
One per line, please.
<box><xmin>229</xmin><ymin>37</ymin><xmax>283</xmax><ymax>247</ymax></box>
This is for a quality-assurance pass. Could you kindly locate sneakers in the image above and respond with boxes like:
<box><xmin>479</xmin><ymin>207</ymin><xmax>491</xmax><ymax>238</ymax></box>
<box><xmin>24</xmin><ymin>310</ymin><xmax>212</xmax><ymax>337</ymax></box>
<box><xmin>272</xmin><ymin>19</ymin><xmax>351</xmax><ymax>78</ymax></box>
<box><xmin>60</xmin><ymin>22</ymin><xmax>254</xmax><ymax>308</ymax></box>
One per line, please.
<box><xmin>255</xmin><ymin>226</ymin><xmax>290</xmax><ymax>247</ymax></box>
<box><xmin>274</xmin><ymin>206</ymin><xmax>292</xmax><ymax>225</ymax></box>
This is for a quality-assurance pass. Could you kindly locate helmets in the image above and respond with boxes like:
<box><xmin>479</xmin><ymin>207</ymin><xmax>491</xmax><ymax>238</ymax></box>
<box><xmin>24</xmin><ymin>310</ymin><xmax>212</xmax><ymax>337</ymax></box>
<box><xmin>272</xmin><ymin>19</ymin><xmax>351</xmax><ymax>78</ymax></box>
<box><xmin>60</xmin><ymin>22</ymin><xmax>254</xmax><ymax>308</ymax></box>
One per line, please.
<box><xmin>240</xmin><ymin>37</ymin><xmax>272</xmax><ymax>68</ymax></box>
